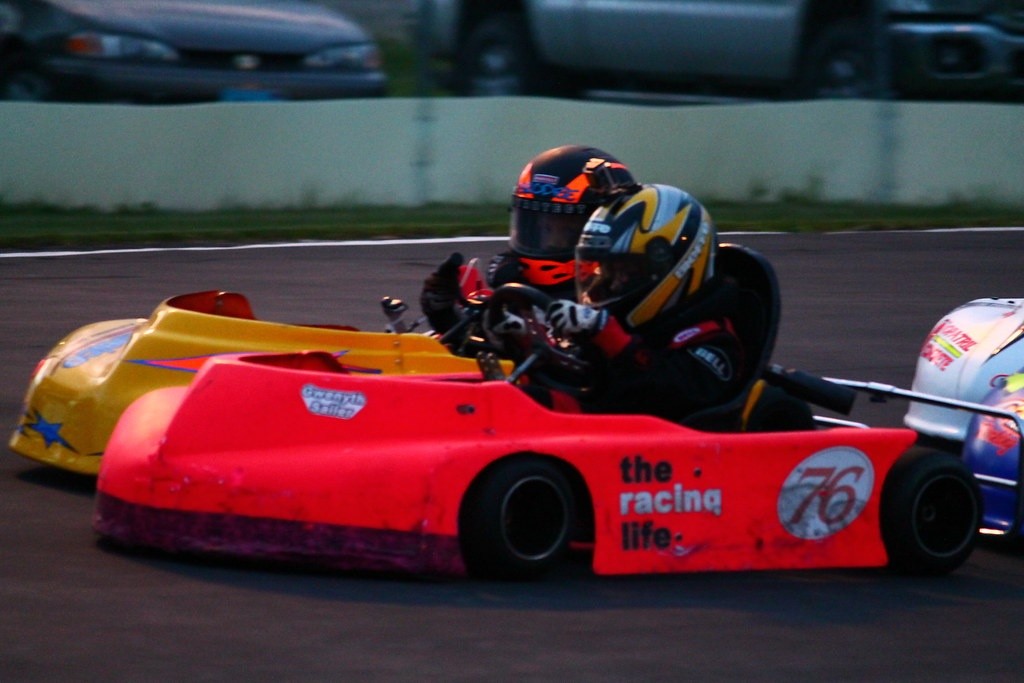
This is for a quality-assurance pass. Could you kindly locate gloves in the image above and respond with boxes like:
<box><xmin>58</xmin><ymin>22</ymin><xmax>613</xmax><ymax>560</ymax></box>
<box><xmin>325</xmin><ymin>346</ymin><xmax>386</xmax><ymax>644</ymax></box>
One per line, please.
<box><xmin>545</xmin><ymin>299</ymin><xmax>608</xmax><ymax>338</ymax></box>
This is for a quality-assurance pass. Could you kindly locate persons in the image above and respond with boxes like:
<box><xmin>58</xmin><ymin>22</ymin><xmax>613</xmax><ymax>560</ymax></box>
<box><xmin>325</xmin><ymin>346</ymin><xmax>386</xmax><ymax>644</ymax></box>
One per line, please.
<box><xmin>419</xmin><ymin>145</ymin><xmax>749</xmax><ymax>432</ymax></box>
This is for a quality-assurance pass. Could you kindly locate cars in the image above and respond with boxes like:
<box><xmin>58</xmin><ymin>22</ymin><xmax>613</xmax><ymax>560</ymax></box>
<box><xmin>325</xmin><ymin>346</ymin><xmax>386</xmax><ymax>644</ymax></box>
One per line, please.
<box><xmin>412</xmin><ymin>0</ymin><xmax>1024</xmax><ymax>107</ymax></box>
<box><xmin>0</xmin><ymin>1</ymin><xmax>389</xmax><ymax>106</ymax></box>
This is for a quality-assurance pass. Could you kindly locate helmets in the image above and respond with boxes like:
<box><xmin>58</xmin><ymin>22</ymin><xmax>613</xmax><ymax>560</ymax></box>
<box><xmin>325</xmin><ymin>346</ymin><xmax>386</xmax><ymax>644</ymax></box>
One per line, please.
<box><xmin>508</xmin><ymin>144</ymin><xmax>637</xmax><ymax>286</ymax></box>
<box><xmin>574</xmin><ymin>183</ymin><xmax>716</xmax><ymax>331</ymax></box>
